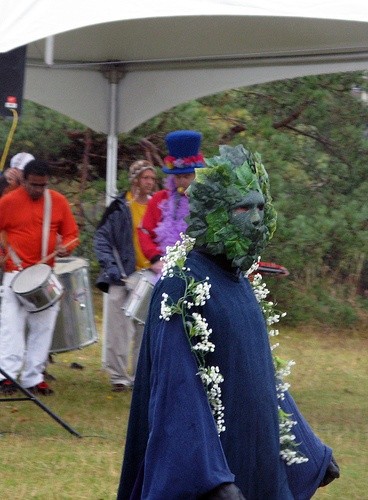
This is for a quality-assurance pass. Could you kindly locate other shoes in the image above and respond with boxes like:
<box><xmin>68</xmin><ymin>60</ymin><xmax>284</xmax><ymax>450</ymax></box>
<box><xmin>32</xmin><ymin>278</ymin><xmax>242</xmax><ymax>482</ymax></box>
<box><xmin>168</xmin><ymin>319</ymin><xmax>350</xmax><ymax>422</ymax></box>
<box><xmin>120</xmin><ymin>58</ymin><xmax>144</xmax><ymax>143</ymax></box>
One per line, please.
<box><xmin>1</xmin><ymin>379</ymin><xmax>17</xmax><ymax>396</ymax></box>
<box><xmin>21</xmin><ymin>381</ymin><xmax>56</xmax><ymax>396</ymax></box>
<box><xmin>111</xmin><ymin>384</ymin><xmax>133</xmax><ymax>393</ymax></box>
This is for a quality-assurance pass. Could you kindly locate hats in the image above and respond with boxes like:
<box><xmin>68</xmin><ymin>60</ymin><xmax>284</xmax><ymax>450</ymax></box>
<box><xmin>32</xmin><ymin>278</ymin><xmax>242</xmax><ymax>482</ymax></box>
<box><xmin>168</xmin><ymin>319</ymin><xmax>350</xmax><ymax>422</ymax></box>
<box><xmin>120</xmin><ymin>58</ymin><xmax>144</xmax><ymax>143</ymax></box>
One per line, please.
<box><xmin>162</xmin><ymin>130</ymin><xmax>203</xmax><ymax>174</ymax></box>
<box><xmin>10</xmin><ymin>153</ymin><xmax>35</xmax><ymax>171</ymax></box>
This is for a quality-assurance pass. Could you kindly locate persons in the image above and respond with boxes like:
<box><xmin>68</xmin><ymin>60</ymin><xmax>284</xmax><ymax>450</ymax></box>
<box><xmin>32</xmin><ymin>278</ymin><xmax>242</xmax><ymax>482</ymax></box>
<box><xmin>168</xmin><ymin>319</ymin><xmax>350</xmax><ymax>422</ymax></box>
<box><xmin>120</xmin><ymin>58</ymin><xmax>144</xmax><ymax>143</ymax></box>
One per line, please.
<box><xmin>1</xmin><ymin>152</ymin><xmax>79</xmax><ymax>395</ymax></box>
<box><xmin>134</xmin><ymin>130</ymin><xmax>220</xmax><ymax>277</ymax></box>
<box><xmin>116</xmin><ymin>144</ymin><xmax>341</xmax><ymax>500</ymax></box>
<box><xmin>93</xmin><ymin>159</ymin><xmax>166</xmax><ymax>392</ymax></box>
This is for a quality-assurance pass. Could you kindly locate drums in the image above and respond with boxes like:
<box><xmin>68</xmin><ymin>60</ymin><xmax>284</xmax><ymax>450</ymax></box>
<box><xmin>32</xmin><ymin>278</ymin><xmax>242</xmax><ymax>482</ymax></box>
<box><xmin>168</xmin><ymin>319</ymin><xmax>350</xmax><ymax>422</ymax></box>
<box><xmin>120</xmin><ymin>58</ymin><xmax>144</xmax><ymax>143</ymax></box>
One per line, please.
<box><xmin>124</xmin><ymin>268</ymin><xmax>161</xmax><ymax>295</ymax></box>
<box><xmin>10</xmin><ymin>262</ymin><xmax>64</xmax><ymax>314</ymax></box>
<box><xmin>48</xmin><ymin>254</ymin><xmax>99</xmax><ymax>355</ymax></box>
<box><xmin>120</xmin><ymin>278</ymin><xmax>156</xmax><ymax>327</ymax></box>
